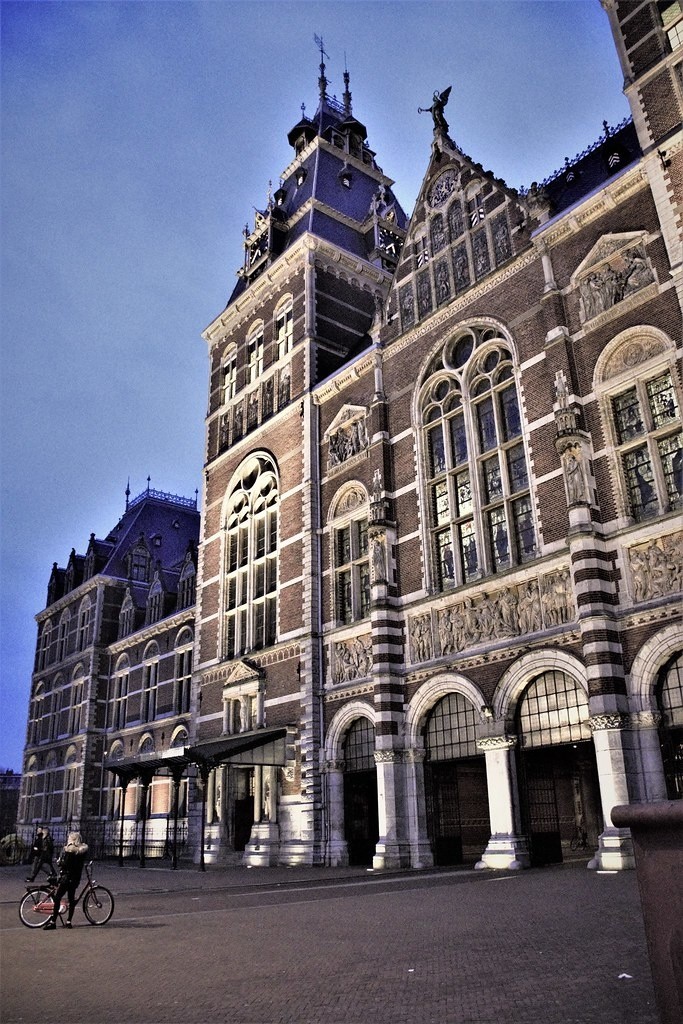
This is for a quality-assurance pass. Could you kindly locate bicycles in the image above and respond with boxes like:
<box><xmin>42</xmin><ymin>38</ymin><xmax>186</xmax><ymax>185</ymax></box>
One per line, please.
<box><xmin>18</xmin><ymin>861</ymin><xmax>115</xmax><ymax>928</ymax></box>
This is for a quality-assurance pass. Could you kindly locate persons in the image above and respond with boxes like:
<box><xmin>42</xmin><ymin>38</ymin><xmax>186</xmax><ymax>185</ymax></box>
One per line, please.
<box><xmin>26</xmin><ymin>828</ymin><xmax>56</xmax><ymax>882</ymax></box>
<box><xmin>240</xmin><ymin>695</ymin><xmax>249</xmax><ymax>732</ymax></box>
<box><xmin>422</xmin><ymin>96</ymin><xmax>453</xmax><ymax>129</ymax></box>
<box><xmin>43</xmin><ymin>832</ymin><xmax>88</xmax><ymax>930</ymax></box>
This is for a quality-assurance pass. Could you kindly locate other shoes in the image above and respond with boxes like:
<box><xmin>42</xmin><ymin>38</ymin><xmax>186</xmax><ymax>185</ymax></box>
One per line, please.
<box><xmin>63</xmin><ymin>922</ymin><xmax>73</xmax><ymax>929</ymax></box>
<box><xmin>43</xmin><ymin>923</ymin><xmax>56</xmax><ymax>930</ymax></box>
<box><xmin>24</xmin><ymin>877</ymin><xmax>33</xmax><ymax>882</ymax></box>
